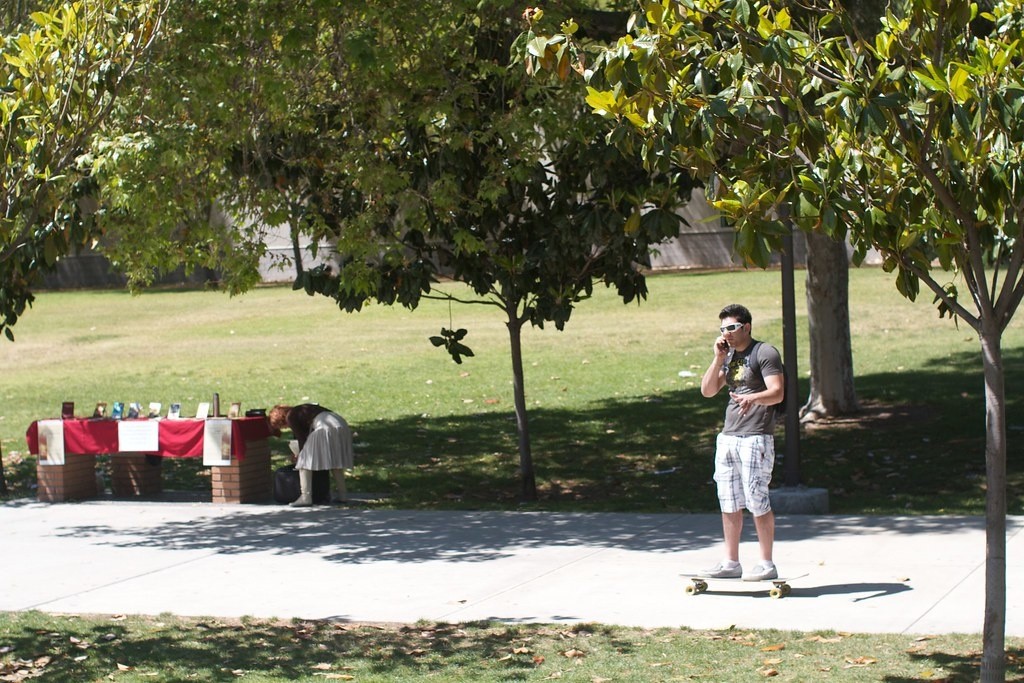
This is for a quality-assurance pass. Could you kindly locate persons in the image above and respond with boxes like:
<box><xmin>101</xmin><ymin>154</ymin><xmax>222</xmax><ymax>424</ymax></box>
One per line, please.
<box><xmin>702</xmin><ymin>304</ymin><xmax>784</xmax><ymax>581</ymax></box>
<box><xmin>270</xmin><ymin>403</ymin><xmax>351</xmax><ymax>506</ymax></box>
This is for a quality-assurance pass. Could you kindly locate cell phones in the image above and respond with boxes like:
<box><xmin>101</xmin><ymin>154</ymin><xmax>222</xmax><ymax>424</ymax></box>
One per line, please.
<box><xmin>722</xmin><ymin>339</ymin><xmax>730</xmax><ymax>350</ymax></box>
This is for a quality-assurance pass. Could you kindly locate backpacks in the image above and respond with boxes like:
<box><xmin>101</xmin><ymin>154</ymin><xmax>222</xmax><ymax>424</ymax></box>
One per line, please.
<box><xmin>750</xmin><ymin>341</ymin><xmax>786</xmax><ymax>414</ymax></box>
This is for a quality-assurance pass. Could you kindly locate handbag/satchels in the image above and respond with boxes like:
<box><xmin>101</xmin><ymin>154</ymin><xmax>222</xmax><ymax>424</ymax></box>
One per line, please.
<box><xmin>273</xmin><ymin>463</ymin><xmax>329</xmax><ymax>504</ymax></box>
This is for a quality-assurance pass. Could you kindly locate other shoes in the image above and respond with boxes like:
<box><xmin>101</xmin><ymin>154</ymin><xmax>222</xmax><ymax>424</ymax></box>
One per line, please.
<box><xmin>706</xmin><ymin>563</ymin><xmax>742</xmax><ymax>578</ymax></box>
<box><xmin>743</xmin><ymin>566</ymin><xmax>778</xmax><ymax>581</ymax></box>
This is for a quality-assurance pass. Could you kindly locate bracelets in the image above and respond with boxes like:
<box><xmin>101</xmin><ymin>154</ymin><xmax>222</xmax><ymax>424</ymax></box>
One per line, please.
<box><xmin>713</xmin><ymin>356</ymin><xmax>725</xmax><ymax>366</ymax></box>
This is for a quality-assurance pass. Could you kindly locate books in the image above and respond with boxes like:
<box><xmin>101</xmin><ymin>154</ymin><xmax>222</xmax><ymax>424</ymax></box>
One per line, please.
<box><xmin>61</xmin><ymin>401</ymin><xmax>240</xmax><ymax>420</ymax></box>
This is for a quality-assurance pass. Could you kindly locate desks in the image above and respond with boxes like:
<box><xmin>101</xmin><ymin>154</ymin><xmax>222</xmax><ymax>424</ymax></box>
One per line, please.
<box><xmin>26</xmin><ymin>416</ymin><xmax>282</xmax><ymax>504</ymax></box>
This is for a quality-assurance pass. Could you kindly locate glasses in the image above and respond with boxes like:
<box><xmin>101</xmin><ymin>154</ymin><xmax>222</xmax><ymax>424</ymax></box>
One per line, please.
<box><xmin>720</xmin><ymin>323</ymin><xmax>743</xmax><ymax>333</ymax></box>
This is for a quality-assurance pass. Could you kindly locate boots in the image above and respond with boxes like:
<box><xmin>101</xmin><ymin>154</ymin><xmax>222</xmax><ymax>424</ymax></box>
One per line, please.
<box><xmin>331</xmin><ymin>469</ymin><xmax>347</xmax><ymax>503</ymax></box>
<box><xmin>289</xmin><ymin>470</ymin><xmax>312</xmax><ymax>507</ymax></box>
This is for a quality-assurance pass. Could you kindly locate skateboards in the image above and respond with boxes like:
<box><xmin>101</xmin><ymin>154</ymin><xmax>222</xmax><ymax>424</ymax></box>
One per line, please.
<box><xmin>678</xmin><ymin>570</ymin><xmax>810</xmax><ymax>599</ymax></box>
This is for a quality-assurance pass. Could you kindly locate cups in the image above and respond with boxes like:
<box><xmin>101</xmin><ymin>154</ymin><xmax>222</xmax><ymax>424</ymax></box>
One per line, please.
<box><xmin>213</xmin><ymin>393</ymin><xmax>219</xmax><ymax>417</ymax></box>
